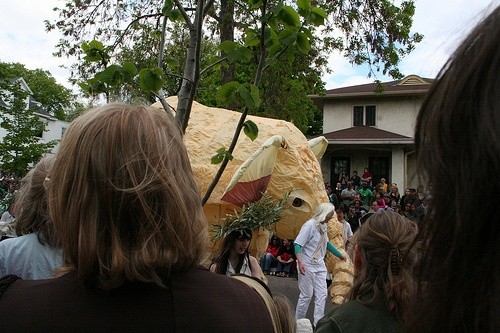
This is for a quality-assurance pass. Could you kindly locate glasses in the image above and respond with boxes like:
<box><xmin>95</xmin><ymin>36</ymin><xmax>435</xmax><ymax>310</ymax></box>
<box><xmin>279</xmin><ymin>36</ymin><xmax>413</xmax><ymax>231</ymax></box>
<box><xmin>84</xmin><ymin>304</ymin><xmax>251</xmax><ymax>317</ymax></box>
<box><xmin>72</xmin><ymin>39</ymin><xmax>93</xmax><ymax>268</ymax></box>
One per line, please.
<box><xmin>358</xmin><ymin>212</ymin><xmax>376</xmax><ymax>228</ymax></box>
<box><xmin>406</xmin><ymin>204</ymin><xmax>411</xmax><ymax>208</ymax></box>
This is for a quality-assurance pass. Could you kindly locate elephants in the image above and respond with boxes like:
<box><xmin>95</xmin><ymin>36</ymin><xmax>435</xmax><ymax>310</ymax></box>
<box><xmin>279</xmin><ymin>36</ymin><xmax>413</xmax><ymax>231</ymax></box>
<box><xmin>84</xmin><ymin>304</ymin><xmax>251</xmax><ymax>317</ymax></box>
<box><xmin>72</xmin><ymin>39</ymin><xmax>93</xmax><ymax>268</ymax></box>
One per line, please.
<box><xmin>148</xmin><ymin>95</ymin><xmax>354</xmax><ymax>306</ymax></box>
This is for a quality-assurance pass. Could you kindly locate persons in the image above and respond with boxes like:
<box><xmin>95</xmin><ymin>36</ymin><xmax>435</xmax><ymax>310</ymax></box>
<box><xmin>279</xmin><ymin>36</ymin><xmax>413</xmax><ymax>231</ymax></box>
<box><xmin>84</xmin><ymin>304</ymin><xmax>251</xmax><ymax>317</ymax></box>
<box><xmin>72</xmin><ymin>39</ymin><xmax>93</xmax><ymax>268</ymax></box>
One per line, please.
<box><xmin>316</xmin><ymin>212</ymin><xmax>419</xmax><ymax>333</ymax></box>
<box><xmin>406</xmin><ymin>9</ymin><xmax>500</xmax><ymax>333</ymax></box>
<box><xmin>0</xmin><ymin>97</ymin><xmax>273</xmax><ymax>333</ymax></box>
<box><xmin>0</xmin><ymin>154</ymin><xmax>427</xmax><ymax>333</ymax></box>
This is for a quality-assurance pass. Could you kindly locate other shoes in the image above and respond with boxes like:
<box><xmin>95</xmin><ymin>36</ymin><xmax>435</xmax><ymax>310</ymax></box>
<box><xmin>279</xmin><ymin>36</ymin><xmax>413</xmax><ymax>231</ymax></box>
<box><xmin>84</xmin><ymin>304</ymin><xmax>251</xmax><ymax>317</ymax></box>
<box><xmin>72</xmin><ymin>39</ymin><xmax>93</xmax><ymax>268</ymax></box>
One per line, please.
<box><xmin>263</xmin><ymin>271</ymin><xmax>267</xmax><ymax>275</ymax></box>
<box><xmin>284</xmin><ymin>274</ymin><xmax>289</xmax><ymax>277</ymax></box>
<box><xmin>276</xmin><ymin>272</ymin><xmax>282</xmax><ymax>277</ymax></box>
